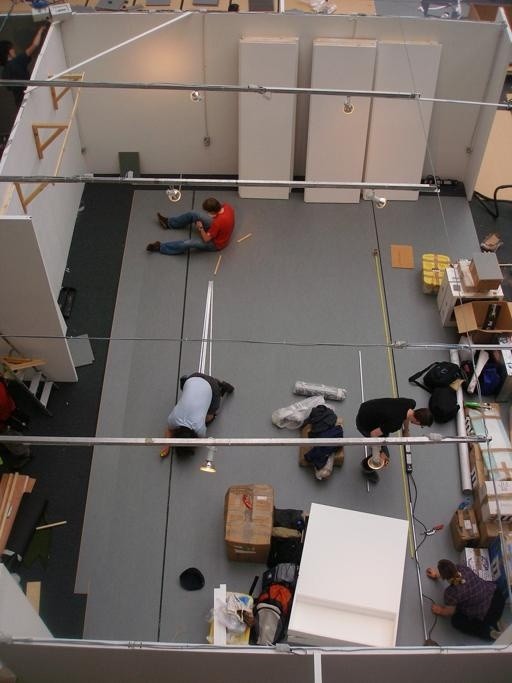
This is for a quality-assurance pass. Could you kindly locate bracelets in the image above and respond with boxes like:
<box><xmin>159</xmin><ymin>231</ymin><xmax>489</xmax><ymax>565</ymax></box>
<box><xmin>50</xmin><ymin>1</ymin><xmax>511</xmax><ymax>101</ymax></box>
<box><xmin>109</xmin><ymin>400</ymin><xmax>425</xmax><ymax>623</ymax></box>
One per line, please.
<box><xmin>197</xmin><ymin>226</ymin><xmax>204</xmax><ymax>233</ymax></box>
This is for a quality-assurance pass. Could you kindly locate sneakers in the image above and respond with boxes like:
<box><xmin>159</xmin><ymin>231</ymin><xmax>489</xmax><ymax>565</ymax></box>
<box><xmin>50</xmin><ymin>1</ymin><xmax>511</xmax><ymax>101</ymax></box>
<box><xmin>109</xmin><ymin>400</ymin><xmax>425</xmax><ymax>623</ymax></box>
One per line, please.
<box><xmin>157</xmin><ymin>212</ymin><xmax>168</xmax><ymax>229</ymax></box>
<box><xmin>490</xmin><ymin>620</ymin><xmax>509</xmax><ymax>640</ymax></box>
<box><xmin>146</xmin><ymin>241</ymin><xmax>161</xmax><ymax>252</ymax></box>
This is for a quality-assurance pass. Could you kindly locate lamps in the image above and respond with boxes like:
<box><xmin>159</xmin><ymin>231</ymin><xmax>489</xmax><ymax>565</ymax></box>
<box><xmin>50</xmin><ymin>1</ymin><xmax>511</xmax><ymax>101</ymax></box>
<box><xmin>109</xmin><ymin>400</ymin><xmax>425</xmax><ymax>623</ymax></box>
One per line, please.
<box><xmin>166</xmin><ymin>185</ymin><xmax>182</xmax><ymax>202</ymax></box>
<box><xmin>199</xmin><ymin>445</ymin><xmax>217</xmax><ymax>473</ymax></box>
<box><xmin>366</xmin><ymin>444</ymin><xmax>384</xmax><ymax>470</ymax></box>
<box><xmin>363</xmin><ymin>188</ymin><xmax>387</xmax><ymax>208</ymax></box>
<box><xmin>342</xmin><ymin>94</ymin><xmax>354</xmax><ymax>114</ymax></box>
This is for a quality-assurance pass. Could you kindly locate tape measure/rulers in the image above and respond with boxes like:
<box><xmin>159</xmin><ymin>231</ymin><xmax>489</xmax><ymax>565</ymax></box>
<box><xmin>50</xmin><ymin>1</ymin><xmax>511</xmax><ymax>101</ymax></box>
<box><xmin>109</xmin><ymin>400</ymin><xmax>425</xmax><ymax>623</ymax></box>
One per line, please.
<box><xmin>373</xmin><ymin>248</ymin><xmax>414</xmax><ymax>560</ymax></box>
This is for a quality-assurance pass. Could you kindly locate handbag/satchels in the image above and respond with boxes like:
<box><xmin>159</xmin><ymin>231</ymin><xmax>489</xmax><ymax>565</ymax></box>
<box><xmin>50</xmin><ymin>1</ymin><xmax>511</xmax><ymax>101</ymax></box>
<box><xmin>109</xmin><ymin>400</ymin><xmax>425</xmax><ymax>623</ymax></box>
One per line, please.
<box><xmin>408</xmin><ymin>361</ymin><xmax>460</xmax><ymax>423</ymax></box>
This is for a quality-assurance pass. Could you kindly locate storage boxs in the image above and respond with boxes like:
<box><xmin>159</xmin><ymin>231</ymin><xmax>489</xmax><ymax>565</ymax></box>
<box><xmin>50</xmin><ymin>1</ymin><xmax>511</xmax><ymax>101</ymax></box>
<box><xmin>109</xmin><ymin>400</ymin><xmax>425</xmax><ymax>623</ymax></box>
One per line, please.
<box><xmin>421</xmin><ymin>252</ymin><xmax>512</xmax><ymax>343</ymax></box>
<box><xmin>449</xmin><ymin>401</ymin><xmax>511</xmax><ymax>612</ymax></box>
<box><xmin>223</xmin><ymin>483</ymin><xmax>275</xmax><ymax>564</ymax></box>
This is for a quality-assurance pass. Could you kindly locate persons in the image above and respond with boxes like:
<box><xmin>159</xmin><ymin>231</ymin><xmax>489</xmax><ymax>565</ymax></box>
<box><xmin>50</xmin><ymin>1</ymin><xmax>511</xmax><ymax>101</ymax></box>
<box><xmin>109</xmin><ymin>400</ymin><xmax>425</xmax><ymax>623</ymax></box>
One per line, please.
<box><xmin>160</xmin><ymin>372</ymin><xmax>235</xmax><ymax>458</ymax></box>
<box><xmin>0</xmin><ymin>383</ymin><xmax>29</xmax><ymax>433</ymax></box>
<box><xmin>426</xmin><ymin>559</ymin><xmax>507</xmax><ymax>641</ymax></box>
<box><xmin>355</xmin><ymin>397</ymin><xmax>434</xmax><ymax>466</ymax></box>
<box><xmin>146</xmin><ymin>196</ymin><xmax>235</xmax><ymax>256</ymax></box>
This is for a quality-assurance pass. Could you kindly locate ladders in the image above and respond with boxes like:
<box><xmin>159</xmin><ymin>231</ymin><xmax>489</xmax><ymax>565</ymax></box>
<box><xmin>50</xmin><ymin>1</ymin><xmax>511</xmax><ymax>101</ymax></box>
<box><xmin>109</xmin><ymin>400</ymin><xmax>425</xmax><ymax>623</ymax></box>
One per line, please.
<box><xmin>0</xmin><ymin>331</ymin><xmax>61</xmax><ymax>417</ymax></box>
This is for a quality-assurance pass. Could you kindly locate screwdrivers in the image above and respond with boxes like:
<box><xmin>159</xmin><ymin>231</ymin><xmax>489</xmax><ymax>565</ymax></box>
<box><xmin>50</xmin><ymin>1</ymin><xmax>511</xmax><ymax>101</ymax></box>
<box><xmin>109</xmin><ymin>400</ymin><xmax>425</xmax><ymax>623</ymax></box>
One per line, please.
<box><xmin>420</xmin><ymin>523</ymin><xmax>444</xmax><ymax>535</ymax></box>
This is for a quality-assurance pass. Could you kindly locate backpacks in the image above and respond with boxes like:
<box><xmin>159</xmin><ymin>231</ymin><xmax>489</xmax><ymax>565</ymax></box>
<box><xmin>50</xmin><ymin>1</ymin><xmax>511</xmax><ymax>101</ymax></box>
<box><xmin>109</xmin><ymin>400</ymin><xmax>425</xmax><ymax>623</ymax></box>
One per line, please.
<box><xmin>250</xmin><ymin>559</ymin><xmax>298</xmax><ymax>644</ymax></box>
